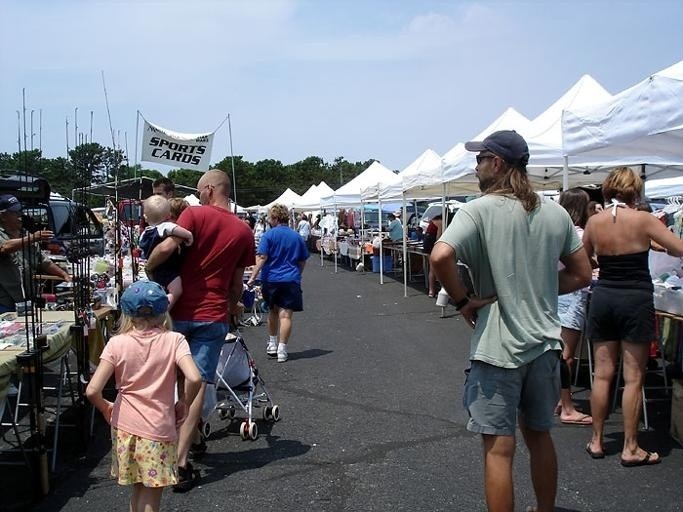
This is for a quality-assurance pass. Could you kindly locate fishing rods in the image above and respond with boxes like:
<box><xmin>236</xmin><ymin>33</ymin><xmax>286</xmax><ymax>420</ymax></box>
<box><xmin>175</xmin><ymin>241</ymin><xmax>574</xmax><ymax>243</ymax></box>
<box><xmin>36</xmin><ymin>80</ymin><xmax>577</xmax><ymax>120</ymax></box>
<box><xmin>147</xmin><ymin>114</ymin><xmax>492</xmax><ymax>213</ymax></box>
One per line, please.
<box><xmin>16</xmin><ymin>88</ymin><xmax>51</xmax><ymax>496</ymax></box>
<box><xmin>100</xmin><ymin>68</ymin><xmax>136</xmax><ymax>333</ymax></box>
<box><xmin>66</xmin><ymin>108</ymin><xmax>93</xmax><ymax>472</ymax></box>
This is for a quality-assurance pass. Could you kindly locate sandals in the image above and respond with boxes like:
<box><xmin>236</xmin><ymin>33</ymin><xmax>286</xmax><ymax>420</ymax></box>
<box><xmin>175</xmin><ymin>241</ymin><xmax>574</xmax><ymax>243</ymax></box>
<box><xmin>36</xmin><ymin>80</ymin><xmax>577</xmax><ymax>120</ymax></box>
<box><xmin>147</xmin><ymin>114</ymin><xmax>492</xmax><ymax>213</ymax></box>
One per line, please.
<box><xmin>173</xmin><ymin>462</ymin><xmax>201</xmax><ymax>492</ymax></box>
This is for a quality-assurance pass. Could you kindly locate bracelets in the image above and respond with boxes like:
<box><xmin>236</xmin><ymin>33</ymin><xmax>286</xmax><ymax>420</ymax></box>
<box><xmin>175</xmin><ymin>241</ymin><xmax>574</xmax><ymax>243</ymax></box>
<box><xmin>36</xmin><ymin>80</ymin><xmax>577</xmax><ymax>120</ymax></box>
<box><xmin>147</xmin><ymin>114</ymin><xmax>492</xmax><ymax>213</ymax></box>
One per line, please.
<box><xmin>454</xmin><ymin>292</ymin><xmax>470</xmax><ymax>311</ymax></box>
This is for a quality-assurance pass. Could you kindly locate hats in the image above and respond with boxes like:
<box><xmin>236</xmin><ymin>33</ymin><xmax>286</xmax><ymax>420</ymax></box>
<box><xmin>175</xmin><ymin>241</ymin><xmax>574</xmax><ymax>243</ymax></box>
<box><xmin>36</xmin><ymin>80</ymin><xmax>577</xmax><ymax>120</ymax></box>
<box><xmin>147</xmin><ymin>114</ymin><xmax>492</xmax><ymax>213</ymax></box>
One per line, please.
<box><xmin>0</xmin><ymin>194</ymin><xmax>23</xmax><ymax>212</ymax></box>
<box><xmin>120</xmin><ymin>280</ymin><xmax>168</xmax><ymax>317</ymax></box>
<box><xmin>465</xmin><ymin>130</ymin><xmax>529</xmax><ymax>166</ymax></box>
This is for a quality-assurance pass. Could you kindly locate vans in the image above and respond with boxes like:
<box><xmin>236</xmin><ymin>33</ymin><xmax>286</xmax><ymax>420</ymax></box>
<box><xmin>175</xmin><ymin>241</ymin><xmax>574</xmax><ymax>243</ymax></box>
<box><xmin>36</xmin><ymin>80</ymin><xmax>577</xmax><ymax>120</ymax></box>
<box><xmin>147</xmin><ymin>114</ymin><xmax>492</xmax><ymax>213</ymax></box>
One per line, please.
<box><xmin>117</xmin><ymin>198</ymin><xmax>145</xmax><ymax>229</ymax></box>
<box><xmin>0</xmin><ymin>169</ymin><xmax>106</xmax><ymax>265</ymax></box>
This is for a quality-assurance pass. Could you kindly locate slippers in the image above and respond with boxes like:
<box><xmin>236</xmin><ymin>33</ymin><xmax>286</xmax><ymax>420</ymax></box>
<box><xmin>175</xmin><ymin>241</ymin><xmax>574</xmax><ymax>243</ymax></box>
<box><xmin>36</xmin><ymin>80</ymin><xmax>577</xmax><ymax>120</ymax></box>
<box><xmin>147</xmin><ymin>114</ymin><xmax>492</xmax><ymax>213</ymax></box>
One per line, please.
<box><xmin>561</xmin><ymin>414</ymin><xmax>593</xmax><ymax>424</ymax></box>
<box><xmin>621</xmin><ymin>450</ymin><xmax>662</xmax><ymax>467</ymax></box>
<box><xmin>586</xmin><ymin>441</ymin><xmax>604</xmax><ymax>458</ymax></box>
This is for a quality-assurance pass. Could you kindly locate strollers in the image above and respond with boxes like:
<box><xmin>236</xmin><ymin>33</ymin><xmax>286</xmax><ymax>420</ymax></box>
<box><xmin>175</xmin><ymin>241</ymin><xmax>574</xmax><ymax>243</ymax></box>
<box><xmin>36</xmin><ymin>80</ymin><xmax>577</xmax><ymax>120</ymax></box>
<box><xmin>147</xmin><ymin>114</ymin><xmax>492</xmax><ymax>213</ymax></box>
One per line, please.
<box><xmin>198</xmin><ymin>310</ymin><xmax>280</xmax><ymax>440</ymax></box>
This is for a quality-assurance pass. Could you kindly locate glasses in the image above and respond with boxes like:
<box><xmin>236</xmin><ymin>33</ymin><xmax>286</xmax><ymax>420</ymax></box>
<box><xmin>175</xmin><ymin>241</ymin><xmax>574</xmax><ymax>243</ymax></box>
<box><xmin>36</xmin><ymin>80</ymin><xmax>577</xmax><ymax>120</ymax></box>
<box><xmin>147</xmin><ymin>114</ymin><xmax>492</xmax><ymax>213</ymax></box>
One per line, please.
<box><xmin>195</xmin><ymin>185</ymin><xmax>214</xmax><ymax>197</ymax></box>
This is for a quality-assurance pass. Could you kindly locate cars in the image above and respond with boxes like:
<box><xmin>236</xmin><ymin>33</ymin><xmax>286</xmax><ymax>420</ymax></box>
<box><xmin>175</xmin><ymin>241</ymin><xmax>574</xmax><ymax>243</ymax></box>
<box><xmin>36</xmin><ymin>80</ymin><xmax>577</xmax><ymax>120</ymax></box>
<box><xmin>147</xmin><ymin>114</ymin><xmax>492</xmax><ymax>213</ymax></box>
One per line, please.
<box><xmin>405</xmin><ymin>206</ymin><xmax>427</xmax><ymax>227</ymax></box>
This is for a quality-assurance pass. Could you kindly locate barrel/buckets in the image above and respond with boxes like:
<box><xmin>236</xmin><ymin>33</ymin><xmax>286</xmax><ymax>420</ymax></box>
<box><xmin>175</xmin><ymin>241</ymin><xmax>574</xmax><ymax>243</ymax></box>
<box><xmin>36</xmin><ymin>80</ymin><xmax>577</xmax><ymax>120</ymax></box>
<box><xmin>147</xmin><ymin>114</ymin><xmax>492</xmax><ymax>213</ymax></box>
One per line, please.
<box><xmin>370</xmin><ymin>255</ymin><xmax>380</xmax><ymax>274</ymax></box>
<box><xmin>383</xmin><ymin>255</ymin><xmax>394</xmax><ymax>273</ymax></box>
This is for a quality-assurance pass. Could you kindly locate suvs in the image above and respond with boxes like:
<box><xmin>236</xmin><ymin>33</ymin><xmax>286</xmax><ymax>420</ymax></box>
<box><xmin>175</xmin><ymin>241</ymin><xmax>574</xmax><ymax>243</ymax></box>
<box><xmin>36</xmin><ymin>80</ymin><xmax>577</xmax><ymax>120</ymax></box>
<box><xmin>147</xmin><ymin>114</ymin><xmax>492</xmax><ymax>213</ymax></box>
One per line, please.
<box><xmin>364</xmin><ymin>211</ymin><xmax>390</xmax><ymax>233</ymax></box>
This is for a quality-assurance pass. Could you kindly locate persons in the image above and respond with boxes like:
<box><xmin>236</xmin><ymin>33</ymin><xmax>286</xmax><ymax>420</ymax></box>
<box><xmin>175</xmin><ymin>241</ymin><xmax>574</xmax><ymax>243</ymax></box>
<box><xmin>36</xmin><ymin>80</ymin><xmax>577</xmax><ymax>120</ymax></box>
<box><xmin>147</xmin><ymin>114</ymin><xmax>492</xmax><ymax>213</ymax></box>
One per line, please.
<box><xmin>552</xmin><ymin>186</ymin><xmax>603</xmax><ymax>426</ymax></box>
<box><xmin>0</xmin><ymin>193</ymin><xmax>74</xmax><ymax>313</ymax></box>
<box><xmin>144</xmin><ymin>168</ymin><xmax>256</xmax><ymax>494</ymax></box>
<box><xmin>243</xmin><ymin>209</ymin><xmax>328</xmax><ymax>247</ymax></box>
<box><xmin>245</xmin><ymin>204</ymin><xmax>311</xmax><ymax>364</ymax></box>
<box><xmin>136</xmin><ymin>176</ymin><xmax>193</xmax><ymax>314</ymax></box>
<box><xmin>581</xmin><ymin>166</ymin><xmax>682</xmax><ymax>467</ymax></box>
<box><xmin>382</xmin><ymin>212</ymin><xmax>407</xmax><ymax>267</ymax></box>
<box><xmin>422</xmin><ymin>213</ymin><xmax>451</xmax><ymax>298</ymax></box>
<box><xmin>81</xmin><ymin>278</ymin><xmax>204</xmax><ymax>511</ymax></box>
<box><xmin>415</xmin><ymin>226</ymin><xmax>425</xmax><ymax>244</ymax></box>
<box><xmin>428</xmin><ymin>129</ymin><xmax>594</xmax><ymax>511</ymax></box>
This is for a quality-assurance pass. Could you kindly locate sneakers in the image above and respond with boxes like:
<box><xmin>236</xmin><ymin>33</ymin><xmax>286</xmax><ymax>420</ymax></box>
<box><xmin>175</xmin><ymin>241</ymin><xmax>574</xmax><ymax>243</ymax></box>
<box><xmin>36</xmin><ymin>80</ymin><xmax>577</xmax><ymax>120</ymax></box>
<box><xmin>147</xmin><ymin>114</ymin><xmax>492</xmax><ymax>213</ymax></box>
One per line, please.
<box><xmin>266</xmin><ymin>342</ymin><xmax>288</xmax><ymax>362</ymax></box>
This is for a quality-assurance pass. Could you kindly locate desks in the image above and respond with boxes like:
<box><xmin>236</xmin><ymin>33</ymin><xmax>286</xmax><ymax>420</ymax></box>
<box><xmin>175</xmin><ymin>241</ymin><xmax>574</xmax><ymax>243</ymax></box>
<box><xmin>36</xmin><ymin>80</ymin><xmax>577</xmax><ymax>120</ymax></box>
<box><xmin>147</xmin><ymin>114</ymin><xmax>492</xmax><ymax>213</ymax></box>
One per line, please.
<box><xmin>1</xmin><ymin>304</ymin><xmax>113</xmax><ymax>498</ymax></box>
